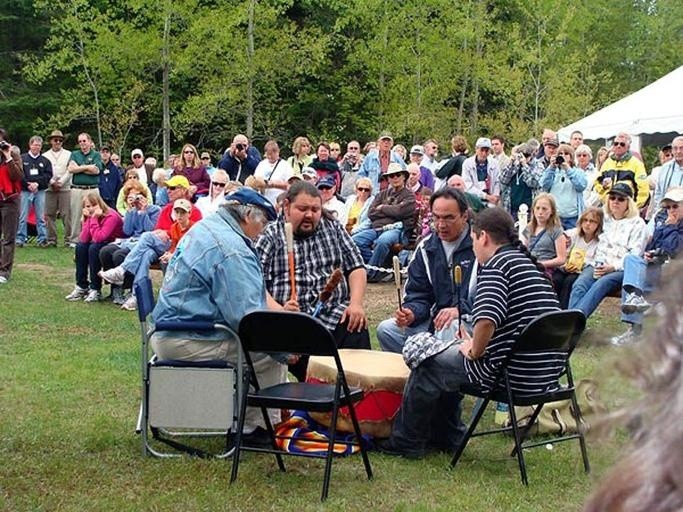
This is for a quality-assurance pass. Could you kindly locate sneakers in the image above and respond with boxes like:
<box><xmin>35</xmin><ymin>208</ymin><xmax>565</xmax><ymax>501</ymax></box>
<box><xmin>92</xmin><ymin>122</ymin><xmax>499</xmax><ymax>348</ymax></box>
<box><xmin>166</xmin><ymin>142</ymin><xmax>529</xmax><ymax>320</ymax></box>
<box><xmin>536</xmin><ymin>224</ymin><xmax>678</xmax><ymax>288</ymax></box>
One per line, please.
<box><xmin>15</xmin><ymin>240</ymin><xmax>71</xmax><ymax>248</ymax></box>
<box><xmin>622</xmin><ymin>292</ymin><xmax>649</xmax><ymax>314</ymax></box>
<box><xmin>226</xmin><ymin>426</ymin><xmax>273</xmax><ymax>450</ymax></box>
<box><xmin>374</xmin><ymin>438</ymin><xmax>400</xmax><ymax>456</ymax></box>
<box><xmin>613</xmin><ymin>329</ymin><xmax>642</xmax><ymax>345</ymax></box>
<box><xmin>0</xmin><ymin>276</ymin><xmax>7</xmax><ymax>283</ymax></box>
<box><xmin>66</xmin><ymin>268</ymin><xmax>138</xmax><ymax>310</ymax></box>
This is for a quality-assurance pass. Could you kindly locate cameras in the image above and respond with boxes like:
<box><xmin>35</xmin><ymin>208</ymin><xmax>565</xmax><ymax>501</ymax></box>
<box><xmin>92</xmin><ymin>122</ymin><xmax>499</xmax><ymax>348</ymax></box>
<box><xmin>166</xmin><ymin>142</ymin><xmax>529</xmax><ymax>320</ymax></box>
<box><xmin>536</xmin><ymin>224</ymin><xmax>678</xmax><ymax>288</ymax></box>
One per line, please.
<box><xmin>517</xmin><ymin>154</ymin><xmax>523</xmax><ymax>159</ymax></box>
<box><xmin>129</xmin><ymin>194</ymin><xmax>142</xmax><ymax>202</ymax></box>
<box><xmin>646</xmin><ymin>246</ymin><xmax>670</xmax><ymax>264</ymax></box>
<box><xmin>235</xmin><ymin>143</ymin><xmax>246</xmax><ymax>151</ymax></box>
<box><xmin>0</xmin><ymin>141</ymin><xmax>8</xmax><ymax>150</ymax></box>
<box><xmin>554</xmin><ymin>154</ymin><xmax>566</xmax><ymax>163</ymax></box>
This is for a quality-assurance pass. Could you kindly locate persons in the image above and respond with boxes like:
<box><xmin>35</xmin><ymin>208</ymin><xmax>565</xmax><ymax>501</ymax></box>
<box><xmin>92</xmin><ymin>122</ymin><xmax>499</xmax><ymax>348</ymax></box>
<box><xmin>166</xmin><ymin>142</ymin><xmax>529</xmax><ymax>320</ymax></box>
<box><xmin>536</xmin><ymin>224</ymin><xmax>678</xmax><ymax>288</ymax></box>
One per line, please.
<box><xmin>98</xmin><ymin>142</ymin><xmax>215</xmax><ymax>209</ymax></box>
<box><xmin>144</xmin><ymin>184</ymin><xmax>301</xmax><ymax>460</ymax></box>
<box><xmin>97</xmin><ymin>174</ymin><xmax>191</xmax><ymax>311</ymax></box>
<box><xmin>376</xmin><ymin>207</ymin><xmax>569</xmax><ymax>458</ymax></box>
<box><xmin>65</xmin><ymin>189</ymin><xmax>125</xmax><ymax>302</ymax></box>
<box><xmin>336</xmin><ymin>133</ymin><xmax>441</xmax><ymax>284</ymax></box>
<box><xmin>193</xmin><ymin>131</ymin><xmax>348</xmax><ymax>229</ymax></box>
<box><xmin>250</xmin><ymin>180</ymin><xmax>371</xmax><ymax>383</ymax></box>
<box><xmin>0</xmin><ymin>129</ymin><xmax>25</xmax><ymax>285</ymax></box>
<box><xmin>158</xmin><ymin>199</ymin><xmax>196</xmax><ymax>276</ymax></box>
<box><xmin>540</xmin><ymin>128</ymin><xmax>683</xmax><ymax>351</ymax></box>
<box><xmin>11</xmin><ymin>129</ymin><xmax>102</xmax><ymax>247</ymax></box>
<box><xmin>376</xmin><ymin>185</ymin><xmax>480</xmax><ymax>352</ymax></box>
<box><xmin>99</xmin><ymin>180</ymin><xmax>162</xmax><ymax>305</ymax></box>
<box><xmin>432</xmin><ymin>128</ymin><xmax>546</xmax><ymax>236</ymax></box>
<box><xmin>573</xmin><ymin>249</ymin><xmax>683</xmax><ymax>511</ymax></box>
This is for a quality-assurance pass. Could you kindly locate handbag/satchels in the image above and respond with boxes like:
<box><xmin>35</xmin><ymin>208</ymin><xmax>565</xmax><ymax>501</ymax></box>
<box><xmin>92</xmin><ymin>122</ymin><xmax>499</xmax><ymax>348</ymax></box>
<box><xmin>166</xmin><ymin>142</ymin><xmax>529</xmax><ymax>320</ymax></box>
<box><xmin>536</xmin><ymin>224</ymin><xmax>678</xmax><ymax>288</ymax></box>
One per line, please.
<box><xmin>503</xmin><ymin>379</ymin><xmax>606</xmax><ymax>436</ymax></box>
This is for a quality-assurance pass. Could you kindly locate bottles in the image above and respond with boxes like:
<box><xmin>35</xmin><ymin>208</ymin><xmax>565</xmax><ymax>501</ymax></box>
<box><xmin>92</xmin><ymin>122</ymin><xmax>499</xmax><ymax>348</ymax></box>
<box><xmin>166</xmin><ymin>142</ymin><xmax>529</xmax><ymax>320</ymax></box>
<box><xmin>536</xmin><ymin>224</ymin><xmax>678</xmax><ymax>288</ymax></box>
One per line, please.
<box><xmin>592</xmin><ymin>251</ymin><xmax>606</xmax><ymax>280</ymax></box>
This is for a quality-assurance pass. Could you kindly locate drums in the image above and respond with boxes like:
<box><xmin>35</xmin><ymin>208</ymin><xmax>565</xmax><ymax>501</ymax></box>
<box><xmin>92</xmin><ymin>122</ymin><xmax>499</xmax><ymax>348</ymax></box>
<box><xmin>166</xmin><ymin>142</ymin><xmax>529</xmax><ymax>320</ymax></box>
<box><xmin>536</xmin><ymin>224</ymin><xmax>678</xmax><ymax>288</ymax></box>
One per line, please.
<box><xmin>303</xmin><ymin>348</ymin><xmax>412</xmax><ymax>438</ymax></box>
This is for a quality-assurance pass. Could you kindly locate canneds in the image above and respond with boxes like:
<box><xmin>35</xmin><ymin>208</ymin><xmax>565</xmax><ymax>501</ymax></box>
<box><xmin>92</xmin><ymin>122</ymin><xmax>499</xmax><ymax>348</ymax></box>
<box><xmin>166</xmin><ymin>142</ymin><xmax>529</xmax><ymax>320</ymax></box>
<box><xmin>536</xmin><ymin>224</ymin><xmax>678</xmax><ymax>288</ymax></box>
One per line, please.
<box><xmin>593</xmin><ymin>261</ymin><xmax>604</xmax><ymax>280</ymax></box>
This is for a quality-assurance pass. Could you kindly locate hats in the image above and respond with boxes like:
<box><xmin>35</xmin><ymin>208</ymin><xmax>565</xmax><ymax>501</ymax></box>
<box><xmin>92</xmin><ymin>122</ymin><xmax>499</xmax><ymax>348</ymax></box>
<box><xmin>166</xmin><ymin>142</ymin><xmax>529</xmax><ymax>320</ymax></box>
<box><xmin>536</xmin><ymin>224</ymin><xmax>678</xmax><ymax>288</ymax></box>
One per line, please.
<box><xmin>402</xmin><ymin>331</ymin><xmax>457</xmax><ymax>369</ymax></box>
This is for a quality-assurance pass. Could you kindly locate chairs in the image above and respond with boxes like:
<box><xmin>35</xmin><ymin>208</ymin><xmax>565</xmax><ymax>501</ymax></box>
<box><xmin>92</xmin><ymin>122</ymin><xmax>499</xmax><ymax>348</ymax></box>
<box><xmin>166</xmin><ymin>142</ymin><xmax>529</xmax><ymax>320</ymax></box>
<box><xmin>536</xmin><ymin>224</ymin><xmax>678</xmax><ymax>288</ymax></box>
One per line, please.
<box><xmin>441</xmin><ymin>310</ymin><xmax>592</xmax><ymax>484</ymax></box>
<box><xmin>390</xmin><ymin>209</ymin><xmax>423</xmax><ymax>251</ymax></box>
<box><xmin>230</xmin><ymin>309</ymin><xmax>373</xmax><ymax>499</ymax></box>
<box><xmin>133</xmin><ymin>278</ymin><xmax>242</xmax><ymax>463</ymax></box>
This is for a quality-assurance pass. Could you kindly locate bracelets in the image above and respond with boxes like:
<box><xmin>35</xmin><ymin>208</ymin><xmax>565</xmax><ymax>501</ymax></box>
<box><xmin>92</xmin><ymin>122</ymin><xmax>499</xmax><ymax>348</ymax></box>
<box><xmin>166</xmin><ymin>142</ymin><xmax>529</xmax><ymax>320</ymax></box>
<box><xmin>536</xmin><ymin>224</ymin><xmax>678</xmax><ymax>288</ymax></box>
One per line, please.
<box><xmin>468</xmin><ymin>348</ymin><xmax>474</xmax><ymax>358</ymax></box>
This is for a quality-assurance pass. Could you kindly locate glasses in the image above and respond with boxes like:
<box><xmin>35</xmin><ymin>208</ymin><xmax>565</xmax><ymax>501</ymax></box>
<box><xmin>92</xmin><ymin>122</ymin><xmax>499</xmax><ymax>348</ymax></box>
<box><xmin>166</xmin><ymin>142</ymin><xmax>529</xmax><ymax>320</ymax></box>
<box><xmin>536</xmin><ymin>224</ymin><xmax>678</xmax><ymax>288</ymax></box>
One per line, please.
<box><xmin>48</xmin><ymin>130</ymin><xmax>683</xmax><ymax>220</ymax></box>
